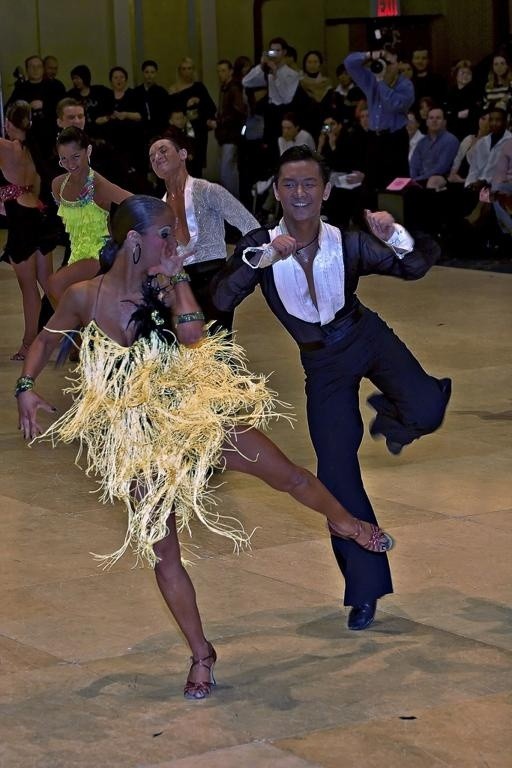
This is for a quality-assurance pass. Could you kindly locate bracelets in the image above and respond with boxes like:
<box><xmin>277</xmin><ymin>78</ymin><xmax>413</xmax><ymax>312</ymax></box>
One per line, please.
<box><xmin>175</xmin><ymin>310</ymin><xmax>206</xmax><ymax>327</ymax></box>
<box><xmin>12</xmin><ymin>376</ymin><xmax>35</xmax><ymax>397</ymax></box>
<box><xmin>170</xmin><ymin>271</ymin><xmax>192</xmax><ymax>287</ymax></box>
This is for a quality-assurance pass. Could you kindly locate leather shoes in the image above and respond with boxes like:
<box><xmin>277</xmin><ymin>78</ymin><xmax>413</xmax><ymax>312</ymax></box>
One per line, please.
<box><xmin>348</xmin><ymin>600</ymin><xmax>377</xmax><ymax>630</ymax></box>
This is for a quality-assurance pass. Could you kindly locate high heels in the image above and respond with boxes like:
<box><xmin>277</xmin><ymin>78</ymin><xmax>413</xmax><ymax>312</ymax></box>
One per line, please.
<box><xmin>327</xmin><ymin>516</ymin><xmax>393</xmax><ymax>553</ymax></box>
<box><xmin>184</xmin><ymin>641</ymin><xmax>216</xmax><ymax>699</ymax></box>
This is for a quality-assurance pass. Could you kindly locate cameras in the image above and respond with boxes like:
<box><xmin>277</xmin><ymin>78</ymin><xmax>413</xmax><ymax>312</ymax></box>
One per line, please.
<box><xmin>370</xmin><ymin>56</ymin><xmax>386</xmax><ymax>75</ymax></box>
<box><xmin>373</xmin><ymin>26</ymin><xmax>393</xmax><ymax>42</ymax></box>
<box><xmin>265</xmin><ymin>48</ymin><xmax>277</xmax><ymax>59</ymax></box>
<box><xmin>322</xmin><ymin>122</ymin><xmax>332</xmax><ymax>135</ymax></box>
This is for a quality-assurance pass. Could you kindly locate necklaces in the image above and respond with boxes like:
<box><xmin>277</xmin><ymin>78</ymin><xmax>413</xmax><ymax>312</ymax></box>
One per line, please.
<box><xmin>292</xmin><ymin>234</ymin><xmax>320</xmax><ymax>256</ymax></box>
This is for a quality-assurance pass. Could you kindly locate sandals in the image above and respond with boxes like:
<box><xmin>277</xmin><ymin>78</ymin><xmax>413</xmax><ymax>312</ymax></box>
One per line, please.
<box><xmin>10</xmin><ymin>340</ymin><xmax>32</xmax><ymax>360</ymax></box>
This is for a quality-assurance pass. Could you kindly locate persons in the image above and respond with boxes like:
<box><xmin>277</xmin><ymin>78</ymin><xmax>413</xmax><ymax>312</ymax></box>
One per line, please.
<box><xmin>13</xmin><ymin>190</ymin><xmax>398</xmax><ymax>700</ymax></box>
<box><xmin>36</xmin><ymin>96</ymin><xmax>106</xmax><ymax>337</ymax></box>
<box><xmin>205</xmin><ymin>143</ymin><xmax>453</xmax><ymax>632</ymax></box>
<box><xmin>342</xmin><ymin>24</ymin><xmax>511</xmax><ymax>270</ymax></box>
<box><xmin>143</xmin><ymin>35</ymin><xmax>350</xmax><ymax>231</ymax></box>
<box><xmin>8</xmin><ymin>53</ymin><xmax>139</xmax><ymax>188</ymax></box>
<box><xmin>40</xmin><ymin>125</ymin><xmax>137</xmax><ymax>311</ymax></box>
<box><xmin>0</xmin><ymin>98</ymin><xmax>70</xmax><ymax>362</ymax></box>
<box><xmin>146</xmin><ymin>134</ymin><xmax>263</xmax><ymax>374</ymax></box>
